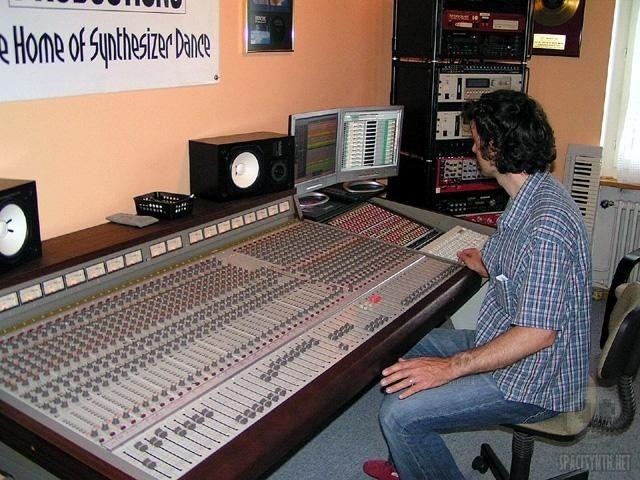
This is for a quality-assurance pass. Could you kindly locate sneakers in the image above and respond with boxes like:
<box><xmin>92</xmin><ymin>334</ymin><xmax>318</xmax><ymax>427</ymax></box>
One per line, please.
<box><xmin>364</xmin><ymin>461</ymin><xmax>400</xmax><ymax>480</ymax></box>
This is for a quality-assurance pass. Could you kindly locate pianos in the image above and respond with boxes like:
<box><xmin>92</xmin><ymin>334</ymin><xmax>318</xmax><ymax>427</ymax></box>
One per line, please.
<box><xmin>563</xmin><ymin>143</ymin><xmax>602</xmax><ymax>247</ymax></box>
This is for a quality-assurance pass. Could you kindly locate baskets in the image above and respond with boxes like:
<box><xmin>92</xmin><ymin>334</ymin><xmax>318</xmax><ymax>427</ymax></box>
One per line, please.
<box><xmin>133</xmin><ymin>192</ymin><xmax>196</xmax><ymax>221</ymax></box>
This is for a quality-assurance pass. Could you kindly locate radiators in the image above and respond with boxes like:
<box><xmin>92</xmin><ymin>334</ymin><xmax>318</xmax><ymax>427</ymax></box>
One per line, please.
<box><xmin>590</xmin><ymin>188</ymin><xmax>640</xmax><ymax>291</ymax></box>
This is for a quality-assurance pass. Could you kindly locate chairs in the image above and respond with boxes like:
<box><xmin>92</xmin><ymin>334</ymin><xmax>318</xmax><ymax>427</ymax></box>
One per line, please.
<box><xmin>471</xmin><ymin>282</ymin><xmax>640</xmax><ymax>479</ymax></box>
<box><xmin>599</xmin><ymin>249</ymin><xmax>640</xmax><ymax>350</ymax></box>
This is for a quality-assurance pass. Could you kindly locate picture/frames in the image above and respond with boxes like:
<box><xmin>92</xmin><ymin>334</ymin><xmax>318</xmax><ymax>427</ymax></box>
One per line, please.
<box><xmin>245</xmin><ymin>0</ymin><xmax>294</xmax><ymax>54</ymax></box>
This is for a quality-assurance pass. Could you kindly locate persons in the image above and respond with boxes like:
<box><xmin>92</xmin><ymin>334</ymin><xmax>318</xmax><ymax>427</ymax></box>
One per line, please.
<box><xmin>363</xmin><ymin>91</ymin><xmax>591</xmax><ymax>480</ymax></box>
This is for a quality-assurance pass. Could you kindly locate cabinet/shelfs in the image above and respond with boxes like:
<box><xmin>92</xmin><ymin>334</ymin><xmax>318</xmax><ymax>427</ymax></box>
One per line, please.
<box><xmin>387</xmin><ymin>1</ymin><xmax>534</xmax><ymax>215</ymax></box>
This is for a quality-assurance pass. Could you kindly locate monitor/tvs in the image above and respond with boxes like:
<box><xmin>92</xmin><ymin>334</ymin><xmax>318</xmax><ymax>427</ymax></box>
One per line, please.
<box><xmin>289</xmin><ymin>108</ymin><xmax>340</xmax><ymax>207</ymax></box>
<box><xmin>340</xmin><ymin>105</ymin><xmax>404</xmax><ymax>193</ymax></box>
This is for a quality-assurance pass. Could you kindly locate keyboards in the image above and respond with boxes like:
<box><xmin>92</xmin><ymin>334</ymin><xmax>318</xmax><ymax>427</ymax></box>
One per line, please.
<box><xmin>418</xmin><ymin>225</ymin><xmax>490</xmax><ymax>267</ymax></box>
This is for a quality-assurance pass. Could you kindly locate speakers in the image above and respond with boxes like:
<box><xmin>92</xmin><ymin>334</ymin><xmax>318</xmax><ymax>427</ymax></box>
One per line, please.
<box><xmin>189</xmin><ymin>132</ymin><xmax>295</xmax><ymax>203</ymax></box>
<box><xmin>0</xmin><ymin>179</ymin><xmax>42</xmax><ymax>276</ymax></box>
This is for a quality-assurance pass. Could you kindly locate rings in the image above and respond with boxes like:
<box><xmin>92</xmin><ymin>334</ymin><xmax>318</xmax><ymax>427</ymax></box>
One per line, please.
<box><xmin>410</xmin><ymin>377</ymin><xmax>414</xmax><ymax>387</ymax></box>
